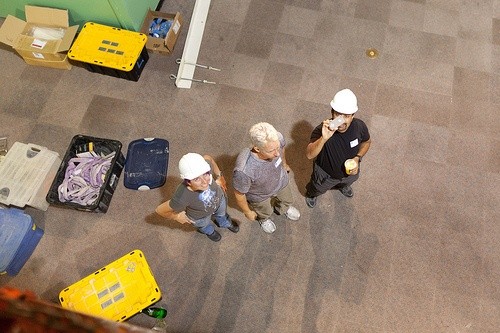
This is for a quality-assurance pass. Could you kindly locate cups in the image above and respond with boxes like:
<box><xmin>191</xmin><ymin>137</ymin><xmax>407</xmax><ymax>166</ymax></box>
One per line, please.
<box><xmin>152</xmin><ymin>320</ymin><xmax>167</xmax><ymax>333</ymax></box>
<box><xmin>344</xmin><ymin>159</ymin><xmax>357</xmax><ymax>175</ymax></box>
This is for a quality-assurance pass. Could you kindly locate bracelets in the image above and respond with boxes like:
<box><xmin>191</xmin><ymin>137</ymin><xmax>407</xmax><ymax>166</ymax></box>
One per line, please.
<box><xmin>215</xmin><ymin>173</ymin><xmax>223</xmax><ymax>178</ymax></box>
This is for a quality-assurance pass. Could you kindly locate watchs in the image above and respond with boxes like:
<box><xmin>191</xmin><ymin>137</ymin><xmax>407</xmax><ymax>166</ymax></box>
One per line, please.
<box><xmin>355</xmin><ymin>155</ymin><xmax>362</xmax><ymax>162</ymax></box>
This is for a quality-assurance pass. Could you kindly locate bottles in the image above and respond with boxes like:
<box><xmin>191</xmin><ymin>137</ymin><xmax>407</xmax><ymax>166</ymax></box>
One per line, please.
<box><xmin>140</xmin><ymin>307</ymin><xmax>167</xmax><ymax>318</ymax></box>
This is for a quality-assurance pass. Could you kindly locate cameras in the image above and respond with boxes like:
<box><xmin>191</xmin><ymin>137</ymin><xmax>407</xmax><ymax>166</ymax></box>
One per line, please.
<box><xmin>328</xmin><ymin>115</ymin><xmax>345</xmax><ymax>131</ymax></box>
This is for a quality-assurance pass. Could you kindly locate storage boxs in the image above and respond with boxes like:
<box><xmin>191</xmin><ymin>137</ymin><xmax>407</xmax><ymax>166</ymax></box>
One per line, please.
<box><xmin>59</xmin><ymin>250</ymin><xmax>163</xmax><ymax>323</ymax></box>
<box><xmin>0</xmin><ymin>208</ymin><xmax>45</xmax><ymax>276</ymax></box>
<box><xmin>0</xmin><ymin>142</ymin><xmax>63</xmax><ymax>212</ymax></box>
<box><xmin>0</xmin><ymin>4</ymin><xmax>80</xmax><ymax>70</ymax></box>
<box><xmin>141</xmin><ymin>8</ymin><xmax>182</xmax><ymax>56</ymax></box>
<box><xmin>45</xmin><ymin>134</ymin><xmax>127</xmax><ymax>214</ymax></box>
<box><xmin>68</xmin><ymin>22</ymin><xmax>149</xmax><ymax>81</ymax></box>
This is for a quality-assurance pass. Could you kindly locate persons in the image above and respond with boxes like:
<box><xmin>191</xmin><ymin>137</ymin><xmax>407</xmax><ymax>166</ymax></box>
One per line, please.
<box><xmin>153</xmin><ymin>152</ymin><xmax>239</xmax><ymax>242</ymax></box>
<box><xmin>304</xmin><ymin>89</ymin><xmax>371</xmax><ymax>207</ymax></box>
<box><xmin>232</xmin><ymin>122</ymin><xmax>301</xmax><ymax>233</ymax></box>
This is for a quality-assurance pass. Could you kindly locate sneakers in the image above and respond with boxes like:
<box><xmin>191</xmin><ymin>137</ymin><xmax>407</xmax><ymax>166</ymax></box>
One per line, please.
<box><xmin>306</xmin><ymin>194</ymin><xmax>317</xmax><ymax>208</ymax></box>
<box><xmin>340</xmin><ymin>185</ymin><xmax>354</xmax><ymax>197</ymax></box>
<box><xmin>260</xmin><ymin>219</ymin><xmax>276</xmax><ymax>233</ymax></box>
<box><xmin>206</xmin><ymin>230</ymin><xmax>221</xmax><ymax>241</ymax></box>
<box><xmin>284</xmin><ymin>206</ymin><xmax>301</xmax><ymax>220</ymax></box>
<box><xmin>227</xmin><ymin>220</ymin><xmax>239</xmax><ymax>233</ymax></box>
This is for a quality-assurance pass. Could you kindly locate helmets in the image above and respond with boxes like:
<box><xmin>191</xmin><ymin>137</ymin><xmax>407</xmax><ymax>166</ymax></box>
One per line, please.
<box><xmin>179</xmin><ymin>152</ymin><xmax>211</xmax><ymax>180</ymax></box>
<box><xmin>330</xmin><ymin>88</ymin><xmax>359</xmax><ymax>114</ymax></box>
